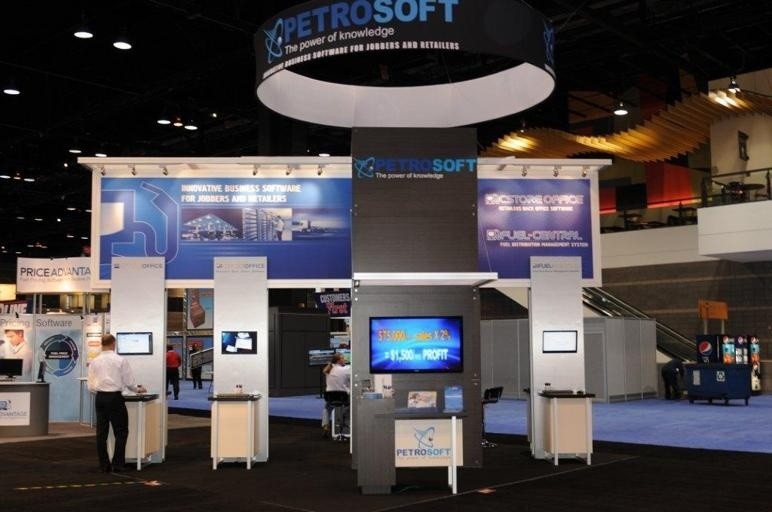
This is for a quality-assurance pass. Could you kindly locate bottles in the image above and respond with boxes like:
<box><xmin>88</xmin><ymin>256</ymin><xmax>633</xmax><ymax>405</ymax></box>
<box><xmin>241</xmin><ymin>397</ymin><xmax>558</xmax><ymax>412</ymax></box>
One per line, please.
<box><xmin>235</xmin><ymin>383</ymin><xmax>242</xmax><ymax>393</ymax></box>
<box><xmin>544</xmin><ymin>383</ymin><xmax>551</xmax><ymax>393</ymax></box>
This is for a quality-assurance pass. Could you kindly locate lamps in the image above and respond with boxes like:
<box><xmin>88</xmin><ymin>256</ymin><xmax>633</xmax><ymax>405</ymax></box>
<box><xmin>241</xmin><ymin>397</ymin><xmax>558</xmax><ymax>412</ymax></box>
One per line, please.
<box><xmin>612</xmin><ymin>99</ymin><xmax>630</xmax><ymax>117</ymax></box>
<box><xmin>725</xmin><ymin>76</ymin><xmax>743</xmax><ymax>95</ymax></box>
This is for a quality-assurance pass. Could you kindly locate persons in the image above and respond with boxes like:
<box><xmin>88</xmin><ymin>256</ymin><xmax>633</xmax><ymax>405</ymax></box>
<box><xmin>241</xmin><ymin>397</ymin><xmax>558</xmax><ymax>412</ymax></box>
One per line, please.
<box><xmin>322</xmin><ymin>353</ymin><xmax>351</xmax><ymax>439</ymax></box>
<box><xmin>662</xmin><ymin>359</ymin><xmax>684</xmax><ymax>400</ymax></box>
<box><xmin>81</xmin><ymin>244</ymin><xmax>91</xmax><ymax>257</ymax></box>
<box><xmin>37</xmin><ymin>344</ymin><xmax>48</xmax><ymax>379</ymax></box>
<box><xmin>409</xmin><ymin>393</ymin><xmax>424</xmax><ymax>407</ymax></box>
<box><xmin>165</xmin><ymin>345</ymin><xmax>181</xmax><ymax>400</ymax></box>
<box><xmin>273</xmin><ymin>215</ymin><xmax>284</xmax><ymax>240</ymax></box>
<box><xmin>189</xmin><ymin>343</ymin><xmax>203</xmax><ymax>389</ymax></box>
<box><xmin>4</xmin><ymin>328</ymin><xmax>33</xmax><ymax>380</ymax></box>
<box><xmin>85</xmin><ymin>334</ymin><xmax>147</xmax><ymax>473</ymax></box>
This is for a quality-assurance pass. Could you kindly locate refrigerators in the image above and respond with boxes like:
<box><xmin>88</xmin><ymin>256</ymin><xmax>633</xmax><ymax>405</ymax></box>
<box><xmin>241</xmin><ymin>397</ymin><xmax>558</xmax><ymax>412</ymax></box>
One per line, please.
<box><xmin>696</xmin><ymin>333</ymin><xmax>761</xmax><ymax>396</ymax></box>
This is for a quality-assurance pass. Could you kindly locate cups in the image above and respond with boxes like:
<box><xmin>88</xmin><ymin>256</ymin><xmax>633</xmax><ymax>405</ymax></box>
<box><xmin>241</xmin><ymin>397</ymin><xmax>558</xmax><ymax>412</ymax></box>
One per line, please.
<box><xmin>382</xmin><ymin>384</ymin><xmax>395</xmax><ymax>398</ymax></box>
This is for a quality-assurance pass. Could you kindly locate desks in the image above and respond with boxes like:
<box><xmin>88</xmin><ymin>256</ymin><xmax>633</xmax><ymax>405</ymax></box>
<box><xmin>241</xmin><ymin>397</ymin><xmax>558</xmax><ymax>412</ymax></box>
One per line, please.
<box><xmin>374</xmin><ymin>407</ymin><xmax>468</xmax><ymax>495</ymax></box>
<box><xmin>109</xmin><ymin>391</ymin><xmax>161</xmax><ymax>476</ymax></box>
<box><xmin>683</xmin><ymin>363</ymin><xmax>754</xmax><ymax>408</ymax></box>
<box><xmin>208</xmin><ymin>393</ymin><xmax>264</xmax><ymax>472</ymax></box>
<box><xmin>538</xmin><ymin>388</ymin><xmax>596</xmax><ymax>470</ymax></box>
<box><xmin>0</xmin><ymin>379</ymin><xmax>52</xmax><ymax>441</ymax></box>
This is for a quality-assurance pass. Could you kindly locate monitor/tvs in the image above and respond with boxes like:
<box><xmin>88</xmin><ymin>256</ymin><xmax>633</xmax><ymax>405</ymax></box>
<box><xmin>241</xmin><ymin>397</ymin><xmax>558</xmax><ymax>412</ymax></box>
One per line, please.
<box><xmin>369</xmin><ymin>315</ymin><xmax>464</xmax><ymax>374</ymax></box>
<box><xmin>0</xmin><ymin>359</ymin><xmax>23</xmax><ymax>378</ymax></box>
<box><xmin>543</xmin><ymin>330</ymin><xmax>577</xmax><ymax>353</ymax></box>
<box><xmin>116</xmin><ymin>332</ymin><xmax>152</xmax><ymax>355</ymax></box>
<box><xmin>614</xmin><ymin>182</ymin><xmax>648</xmax><ymax>210</ymax></box>
<box><xmin>221</xmin><ymin>331</ymin><xmax>257</xmax><ymax>355</ymax></box>
<box><xmin>36</xmin><ymin>361</ymin><xmax>46</xmax><ymax>383</ymax></box>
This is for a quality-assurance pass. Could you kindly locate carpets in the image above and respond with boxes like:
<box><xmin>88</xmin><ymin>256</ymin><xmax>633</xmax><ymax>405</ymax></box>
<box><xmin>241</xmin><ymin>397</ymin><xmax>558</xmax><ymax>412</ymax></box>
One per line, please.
<box><xmin>1</xmin><ymin>411</ymin><xmax>211</xmax><ymax>450</ymax></box>
<box><xmin>168</xmin><ymin>380</ymin><xmax>771</xmax><ymax>452</ymax></box>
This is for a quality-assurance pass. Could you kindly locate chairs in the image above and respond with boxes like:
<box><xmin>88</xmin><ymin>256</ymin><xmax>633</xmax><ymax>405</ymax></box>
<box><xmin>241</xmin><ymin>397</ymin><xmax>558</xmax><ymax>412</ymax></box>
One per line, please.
<box><xmin>322</xmin><ymin>390</ymin><xmax>353</xmax><ymax>444</ymax></box>
<box><xmin>482</xmin><ymin>385</ymin><xmax>508</xmax><ymax>449</ymax></box>
